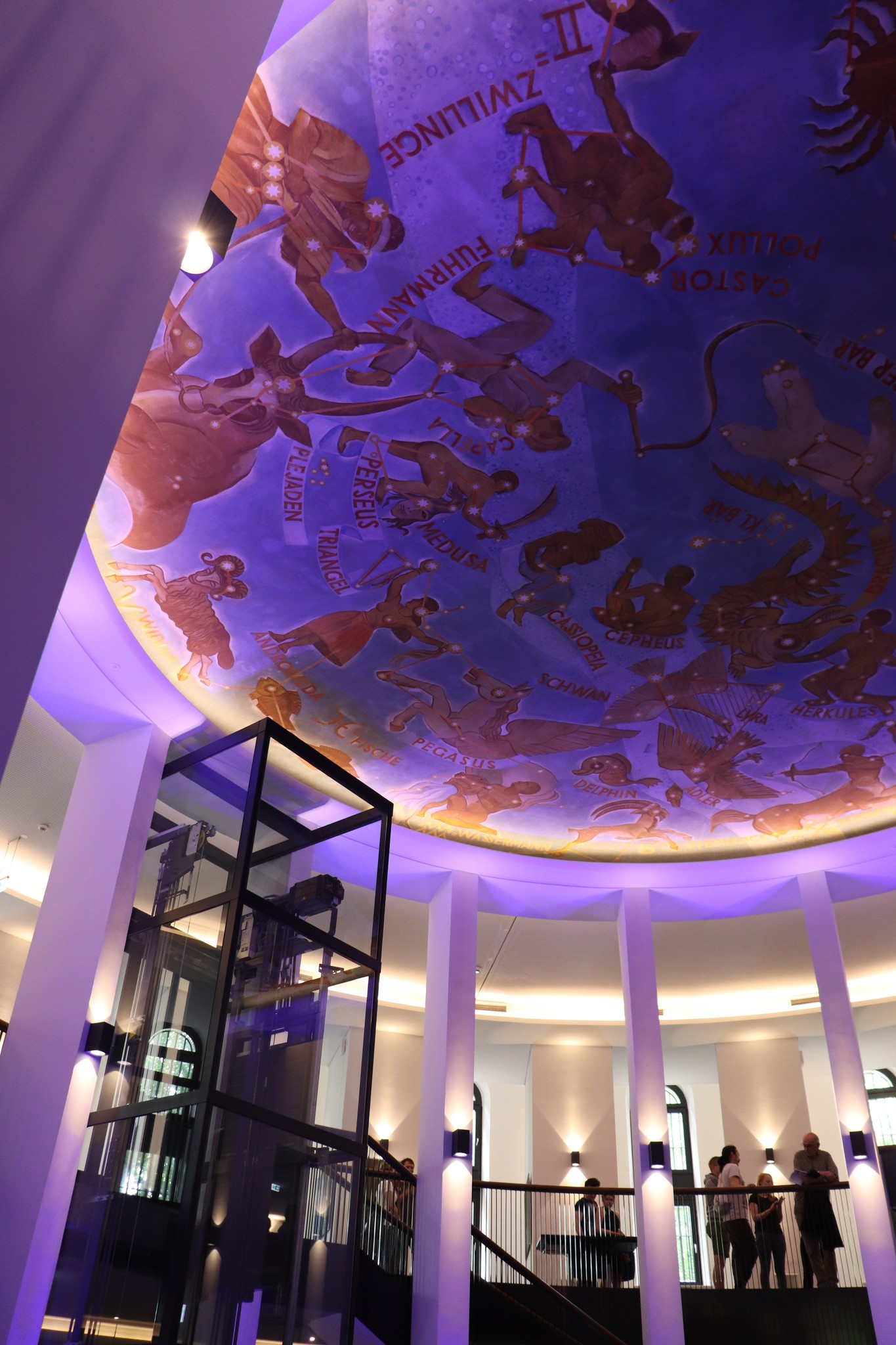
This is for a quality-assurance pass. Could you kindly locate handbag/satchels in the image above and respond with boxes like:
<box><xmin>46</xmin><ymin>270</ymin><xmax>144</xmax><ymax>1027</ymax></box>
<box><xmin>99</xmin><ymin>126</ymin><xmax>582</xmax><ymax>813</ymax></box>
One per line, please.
<box><xmin>621</xmin><ymin>1252</ymin><xmax>635</xmax><ymax>1282</ymax></box>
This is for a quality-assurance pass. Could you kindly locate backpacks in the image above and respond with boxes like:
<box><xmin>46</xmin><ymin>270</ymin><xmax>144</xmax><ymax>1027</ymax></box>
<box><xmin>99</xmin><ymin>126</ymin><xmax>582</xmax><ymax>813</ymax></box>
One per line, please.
<box><xmin>714</xmin><ymin>1173</ymin><xmax>734</xmax><ymax>1216</ymax></box>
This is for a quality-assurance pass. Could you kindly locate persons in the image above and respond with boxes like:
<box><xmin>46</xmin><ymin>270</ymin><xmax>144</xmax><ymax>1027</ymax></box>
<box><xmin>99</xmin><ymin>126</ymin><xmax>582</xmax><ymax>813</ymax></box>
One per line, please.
<box><xmin>715</xmin><ymin>1145</ymin><xmax>758</xmax><ymax>1291</ymax></box>
<box><xmin>788</xmin><ymin>1132</ymin><xmax>844</xmax><ymax>1286</ymax></box>
<box><xmin>704</xmin><ymin>1157</ymin><xmax>730</xmax><ymax>1290</ymax></box>
<box><xmin>385</xmin><ymin>1157</ymin><xmax>417</xmax><ymax>1277</ymax></box>
<box><xmin>749</xmin><ymin>1172</ymin><xmax>787</xmax><ymax>1291</ymax></box>
<box><xmin>575</xmin><ymin>1177</ymin><xmax>600</xmax><ymax>1289</ymax></box>
<box><xmin>596</xmin><ymin>1195</ymin><xmax>625</xmax><ymax>1289</ymax></box>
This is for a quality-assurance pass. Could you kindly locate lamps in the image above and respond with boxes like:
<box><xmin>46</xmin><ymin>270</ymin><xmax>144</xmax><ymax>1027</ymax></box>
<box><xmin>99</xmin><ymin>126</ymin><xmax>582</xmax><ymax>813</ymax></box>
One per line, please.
<box><xmin>765</xmin><ymin>1148</ymin><xmax>775</xmax><ymax>1164</ymax></box>
<box><xmin>849</xmin><ymin>1130</ymin><xmax>868</xmax><ymax>1160</ymax></box>
<box><xmin>571</xmin><ymin>1151</ymin><xmax>580</xmax><ymax>1167</ymax></box>
<box><xmin>451</xmin><ymin>1129</ymin><xmax>470</xmax><ymax>1157</ymax></box>
<box><xmin>379</xmin><ymin>1139</ymin><xmax>389</xmax><ymax>1152</ymax></box>
<box><xmin>180</xmin><ymin>189</ymin><xmax>239</xmax><ymax>282</ymax></box>
<box><xmin>84</xmin><ymin>1020</ymin><xmax>116</xmax><ymax>1059</ymax></box>
<box><xmin>648</xmin><ymin>1142</ymin><xmax>664</xmax><ymax>1170</ymax></box>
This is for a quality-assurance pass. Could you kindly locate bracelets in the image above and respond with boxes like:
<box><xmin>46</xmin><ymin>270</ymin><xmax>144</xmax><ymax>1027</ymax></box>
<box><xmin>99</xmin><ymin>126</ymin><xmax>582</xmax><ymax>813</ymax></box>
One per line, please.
<box><xmin>817</xmin><ymin>1171</ymin><xmax>819</xmax><ymax>1174</ymax></box>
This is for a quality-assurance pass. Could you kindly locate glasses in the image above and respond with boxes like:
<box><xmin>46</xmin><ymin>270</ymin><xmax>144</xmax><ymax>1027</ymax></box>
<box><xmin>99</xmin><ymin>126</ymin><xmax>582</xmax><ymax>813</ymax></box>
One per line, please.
<box><xmin>800</xmin><ymin>1142</ymin><xmax>817</xmax><ymax>1149</ymax></box>
